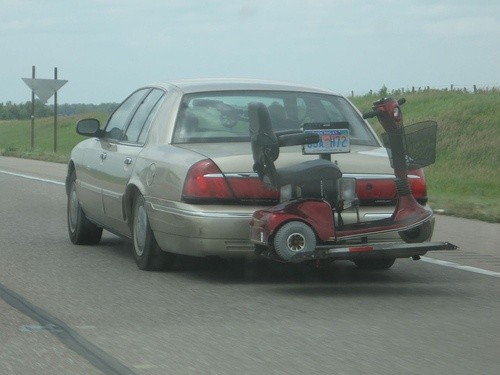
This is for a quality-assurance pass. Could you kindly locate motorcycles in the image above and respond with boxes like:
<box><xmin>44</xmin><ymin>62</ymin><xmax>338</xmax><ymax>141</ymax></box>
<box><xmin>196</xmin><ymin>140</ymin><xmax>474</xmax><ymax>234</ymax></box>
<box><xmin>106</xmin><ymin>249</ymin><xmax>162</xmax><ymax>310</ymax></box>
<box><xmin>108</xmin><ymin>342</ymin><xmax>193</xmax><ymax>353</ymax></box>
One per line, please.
<box><xmin>248</xmin><ymin>97</ymin><xmax>460</xmax><ymax>279</ymax></box>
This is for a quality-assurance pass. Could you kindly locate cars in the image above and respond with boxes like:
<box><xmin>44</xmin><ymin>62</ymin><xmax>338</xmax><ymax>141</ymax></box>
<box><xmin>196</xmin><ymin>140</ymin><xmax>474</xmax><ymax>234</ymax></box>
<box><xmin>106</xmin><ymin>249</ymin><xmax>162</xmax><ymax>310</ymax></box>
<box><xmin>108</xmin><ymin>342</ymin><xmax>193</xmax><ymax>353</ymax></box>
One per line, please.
<box><xmin>65</xmin><ymin>77</ymin><xmax>435</xmax><ymax>270</ymax></box>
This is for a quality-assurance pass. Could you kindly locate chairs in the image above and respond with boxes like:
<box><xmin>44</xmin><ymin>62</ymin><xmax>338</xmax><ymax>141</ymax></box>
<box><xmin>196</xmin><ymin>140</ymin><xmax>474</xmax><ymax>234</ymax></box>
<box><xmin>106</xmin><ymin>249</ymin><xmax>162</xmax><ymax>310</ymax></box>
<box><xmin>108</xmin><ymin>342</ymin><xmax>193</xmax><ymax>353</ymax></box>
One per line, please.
<box><xmin>182</xmin><ymin>110</ymin><xmax>209</xmax><ymax>132</ymax></box>
<box><xmin>269</xmin><ymin>103</ymin><xmax>301</xmax><ymax>131</ymax></box>
